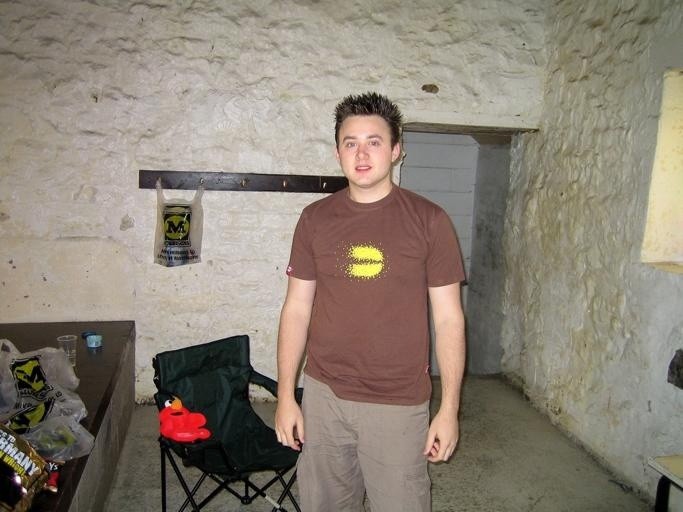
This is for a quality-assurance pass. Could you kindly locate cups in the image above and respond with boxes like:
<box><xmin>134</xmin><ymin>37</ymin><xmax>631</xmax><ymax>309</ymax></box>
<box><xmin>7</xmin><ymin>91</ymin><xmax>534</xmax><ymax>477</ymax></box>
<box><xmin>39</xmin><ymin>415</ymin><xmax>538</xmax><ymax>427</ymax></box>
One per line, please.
<box><xmin>57</xmin><ymin>334</ymin><xmax>78</xmax><ymax>367</ymax></box>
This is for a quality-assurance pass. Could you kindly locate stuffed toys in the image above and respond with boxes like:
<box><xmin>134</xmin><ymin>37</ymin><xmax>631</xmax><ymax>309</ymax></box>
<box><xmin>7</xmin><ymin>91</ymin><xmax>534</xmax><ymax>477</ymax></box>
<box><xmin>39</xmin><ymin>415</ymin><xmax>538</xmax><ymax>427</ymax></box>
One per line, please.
<box><xmin>156</xmin><ymin>399</ymin><xmax>210</xmax><ymax>443</ymax></box>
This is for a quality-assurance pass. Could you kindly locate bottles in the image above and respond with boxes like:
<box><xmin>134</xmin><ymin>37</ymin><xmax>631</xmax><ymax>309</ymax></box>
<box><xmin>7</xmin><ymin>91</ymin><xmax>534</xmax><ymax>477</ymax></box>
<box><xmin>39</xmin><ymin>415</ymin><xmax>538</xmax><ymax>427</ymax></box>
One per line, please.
<box><xmin>86</xmin><ymin>335</ymin><xmax>102</xmax><ymax>347</ymax></box>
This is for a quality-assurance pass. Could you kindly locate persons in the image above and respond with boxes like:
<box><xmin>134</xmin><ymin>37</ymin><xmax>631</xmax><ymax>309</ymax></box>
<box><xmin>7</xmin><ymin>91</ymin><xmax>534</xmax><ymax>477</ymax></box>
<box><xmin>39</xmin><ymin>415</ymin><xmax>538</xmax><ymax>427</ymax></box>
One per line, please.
<box><xmin>274</xmin><ymin>91</ymin><xmax>466</xmax><ymax>512</ymax></box>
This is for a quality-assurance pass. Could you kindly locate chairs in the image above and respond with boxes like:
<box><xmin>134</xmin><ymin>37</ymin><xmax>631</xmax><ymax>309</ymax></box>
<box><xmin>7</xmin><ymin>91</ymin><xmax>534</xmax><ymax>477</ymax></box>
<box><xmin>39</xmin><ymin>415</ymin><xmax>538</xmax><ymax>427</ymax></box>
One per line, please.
<box><xmin>150</xmin><ymin>334</ymin><xmax>304</xmax><ymax>511</ymax></box>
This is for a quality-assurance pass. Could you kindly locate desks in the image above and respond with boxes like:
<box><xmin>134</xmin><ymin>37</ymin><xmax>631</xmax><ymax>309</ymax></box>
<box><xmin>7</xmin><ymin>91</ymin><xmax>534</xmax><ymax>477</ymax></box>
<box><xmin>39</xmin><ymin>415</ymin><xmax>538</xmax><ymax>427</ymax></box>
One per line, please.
<box><xmin>2</xmin><ymin>319</ymin><xmax>138</xmax><ymax>512</ymax></box>
